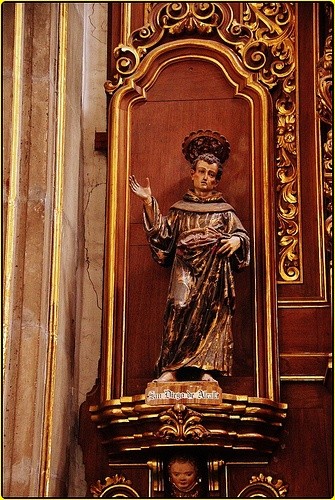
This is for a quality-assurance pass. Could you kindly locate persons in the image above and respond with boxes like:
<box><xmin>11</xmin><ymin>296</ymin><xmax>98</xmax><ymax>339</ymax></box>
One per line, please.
<box><xmin>129</xmin><ymin>154</ymin><xmax>251</xmax><ymax>383</ymax></box>
<box><xmin>168</xmin><ymin>456</ymin><xmax>202</xmax><ymax>498</ymax></box>
